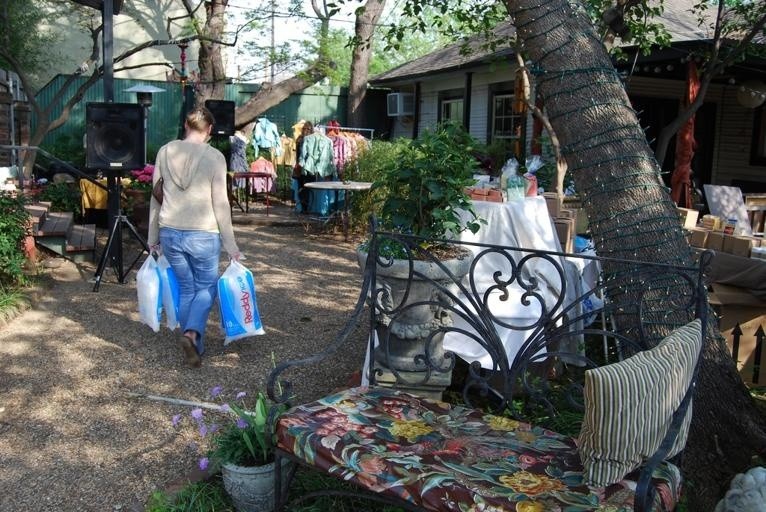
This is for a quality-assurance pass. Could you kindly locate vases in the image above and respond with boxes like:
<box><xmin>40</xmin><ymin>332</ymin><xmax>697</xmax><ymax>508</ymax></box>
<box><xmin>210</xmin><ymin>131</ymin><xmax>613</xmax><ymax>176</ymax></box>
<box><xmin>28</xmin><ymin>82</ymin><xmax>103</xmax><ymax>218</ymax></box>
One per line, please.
<box><xmin>221</xmin><ymin>456</ymin><xmax>296</xmax><ymax>511</ymax></box>
<box><xmin>125</xmin><ymin>189</ymin><xmax>151</xmax><ymax>204</ymax></box>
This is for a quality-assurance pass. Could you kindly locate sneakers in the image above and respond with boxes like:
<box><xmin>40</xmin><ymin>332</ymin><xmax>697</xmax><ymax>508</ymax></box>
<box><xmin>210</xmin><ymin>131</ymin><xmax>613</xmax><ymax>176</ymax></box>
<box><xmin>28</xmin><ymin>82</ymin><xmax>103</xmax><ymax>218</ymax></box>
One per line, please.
<box><xmin>179</xmin><ymin>334</ymin><xmax>202</xmax><ymax>367</ymax></box>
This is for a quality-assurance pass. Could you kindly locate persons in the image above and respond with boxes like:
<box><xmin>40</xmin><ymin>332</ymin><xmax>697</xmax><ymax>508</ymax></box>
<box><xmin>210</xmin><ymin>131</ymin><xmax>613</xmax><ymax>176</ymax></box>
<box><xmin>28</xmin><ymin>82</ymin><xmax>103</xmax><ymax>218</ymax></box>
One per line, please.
<box><xmin>294</xmin><ymin>121</ymin><xmax>319</xmax><ymax>216</ymax></box>
<box><xmin>146</xmin><ymin>105</ymin><xmax>239</xmax><ymax>368</ymax></box>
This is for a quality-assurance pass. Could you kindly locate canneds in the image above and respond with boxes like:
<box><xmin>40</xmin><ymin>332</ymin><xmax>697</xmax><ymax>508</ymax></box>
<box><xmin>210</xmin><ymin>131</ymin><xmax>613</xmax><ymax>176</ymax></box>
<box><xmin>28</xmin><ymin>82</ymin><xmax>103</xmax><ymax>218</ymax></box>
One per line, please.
<box><xmin>723</xmin><ymin>219</ymin><xmax>738</xmax><ymax>235</ymax></box>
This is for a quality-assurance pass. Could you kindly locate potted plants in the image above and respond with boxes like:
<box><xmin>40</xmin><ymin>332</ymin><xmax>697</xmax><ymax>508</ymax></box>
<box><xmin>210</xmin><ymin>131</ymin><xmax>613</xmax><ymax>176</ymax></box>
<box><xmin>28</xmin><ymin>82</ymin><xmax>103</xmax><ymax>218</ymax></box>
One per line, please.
<box><xmin>355</xmin><ymin>118</ymin><xmax>489</xmax><ymax>370</ymax></box>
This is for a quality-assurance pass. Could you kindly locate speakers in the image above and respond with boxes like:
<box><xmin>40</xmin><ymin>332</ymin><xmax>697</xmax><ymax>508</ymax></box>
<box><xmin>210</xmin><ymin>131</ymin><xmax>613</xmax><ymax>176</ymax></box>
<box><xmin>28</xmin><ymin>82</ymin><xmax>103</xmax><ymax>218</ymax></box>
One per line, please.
<box><xmin>204</xmin><ymin>99</ymin><xmax>235</xmax><ymax>137</ymax></box>
<box><xmin>85</xmin><ymin>100</ymin><xmax>147</xmax><ymax>170</ymax></box>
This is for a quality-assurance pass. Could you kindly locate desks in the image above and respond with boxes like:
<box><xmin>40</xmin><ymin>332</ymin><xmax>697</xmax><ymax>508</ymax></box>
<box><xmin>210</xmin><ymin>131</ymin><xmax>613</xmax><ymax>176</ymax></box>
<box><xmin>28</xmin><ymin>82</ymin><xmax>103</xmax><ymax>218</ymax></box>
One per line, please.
<box><xmin>227</xmin><ymin>171</ymin><xmax>272</xmax><ymax>218</ymax></box>
<box><xmin>303</xmin><ymin>181</ymin><xmax>374</xmax><ymax>243</ymax></box>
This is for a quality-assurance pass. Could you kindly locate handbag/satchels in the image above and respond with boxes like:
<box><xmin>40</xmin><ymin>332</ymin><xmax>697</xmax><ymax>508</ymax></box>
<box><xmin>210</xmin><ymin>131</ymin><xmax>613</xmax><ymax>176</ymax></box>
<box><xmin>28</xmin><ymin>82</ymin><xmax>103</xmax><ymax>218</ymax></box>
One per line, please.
<box><xmin>153</xmin><ymin>176</ymin><xmax>164</xmax><ymax>205</ymax></box>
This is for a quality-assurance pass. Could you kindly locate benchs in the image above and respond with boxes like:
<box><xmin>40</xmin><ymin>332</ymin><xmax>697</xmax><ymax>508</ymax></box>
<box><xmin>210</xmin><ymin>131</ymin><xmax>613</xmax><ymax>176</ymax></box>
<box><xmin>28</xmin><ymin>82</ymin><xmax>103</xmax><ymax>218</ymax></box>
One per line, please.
<box><xmin>264</xmin><ymin>231</ymin><xmax>716</xmax><ymax>511</ymax></box>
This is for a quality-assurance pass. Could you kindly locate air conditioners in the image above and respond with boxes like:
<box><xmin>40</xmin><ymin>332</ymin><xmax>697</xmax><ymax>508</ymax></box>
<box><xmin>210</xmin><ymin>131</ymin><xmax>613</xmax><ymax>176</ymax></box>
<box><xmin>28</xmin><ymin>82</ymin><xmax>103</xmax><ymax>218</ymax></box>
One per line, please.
<box><xmin>387</xmin><ymin>93</ymin><xmax>415</xmax><ymax>117</ymax></box>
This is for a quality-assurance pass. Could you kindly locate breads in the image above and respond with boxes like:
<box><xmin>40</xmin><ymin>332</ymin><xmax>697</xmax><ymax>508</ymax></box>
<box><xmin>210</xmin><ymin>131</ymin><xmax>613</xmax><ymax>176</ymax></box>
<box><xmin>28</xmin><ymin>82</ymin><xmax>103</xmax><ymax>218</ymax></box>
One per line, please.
<box><xmin>701</xmin><ymin>215</ymin><xmax>721</xmax><ymax>231</ymax></box>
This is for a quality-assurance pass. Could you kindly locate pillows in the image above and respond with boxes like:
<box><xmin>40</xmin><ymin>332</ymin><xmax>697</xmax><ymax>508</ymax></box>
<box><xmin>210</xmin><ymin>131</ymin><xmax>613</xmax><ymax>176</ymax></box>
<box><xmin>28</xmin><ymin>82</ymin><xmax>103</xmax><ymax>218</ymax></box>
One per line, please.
<box><xmin>577</xmin><ymin>318</ymin><xmax>703</xmax><ymax>486</ymax></box>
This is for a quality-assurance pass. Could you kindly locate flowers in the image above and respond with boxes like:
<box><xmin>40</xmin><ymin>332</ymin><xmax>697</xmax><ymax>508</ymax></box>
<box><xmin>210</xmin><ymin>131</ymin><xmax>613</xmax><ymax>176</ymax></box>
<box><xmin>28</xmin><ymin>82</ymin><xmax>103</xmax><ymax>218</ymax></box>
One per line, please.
<box><xmin>125</xmin><ymin>163</ymin><xmax>155</xmax><ymax>201</ymax></box>
<box><xmin>172</xmin><ymin>384</ymin><xmax>296</xmax><ymax>471</ymax></box>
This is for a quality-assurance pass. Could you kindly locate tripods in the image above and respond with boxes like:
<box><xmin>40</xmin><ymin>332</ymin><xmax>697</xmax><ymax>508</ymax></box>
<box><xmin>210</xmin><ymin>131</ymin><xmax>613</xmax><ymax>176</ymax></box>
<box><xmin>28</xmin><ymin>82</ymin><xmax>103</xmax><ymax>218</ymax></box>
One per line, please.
<box><xmin>215</xmin><ymin>140</ymin><xmax>245</xmax><ymax>213</ymax></box>
<box><xmin>93</xmin><ymin>171</ymin><xmax>152</xmax><ymax>294</ymax></box>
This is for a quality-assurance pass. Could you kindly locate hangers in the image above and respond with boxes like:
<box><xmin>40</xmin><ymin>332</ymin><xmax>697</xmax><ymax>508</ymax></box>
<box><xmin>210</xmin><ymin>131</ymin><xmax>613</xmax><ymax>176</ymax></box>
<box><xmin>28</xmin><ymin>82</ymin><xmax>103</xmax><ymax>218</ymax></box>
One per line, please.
<box><xmin>252</xmin><ymin>156</ymin><xmax>271</xmax><ymax>164</ymax></box>
<box><xmin>304</xmin><ymin>125</ymin><xmax>368</xmax><ymax>142</ymax></box>
<box><xmin>291</xmin><ymin>119</ymin><xmax>306</xmax><ymax>129</ymax></box>
